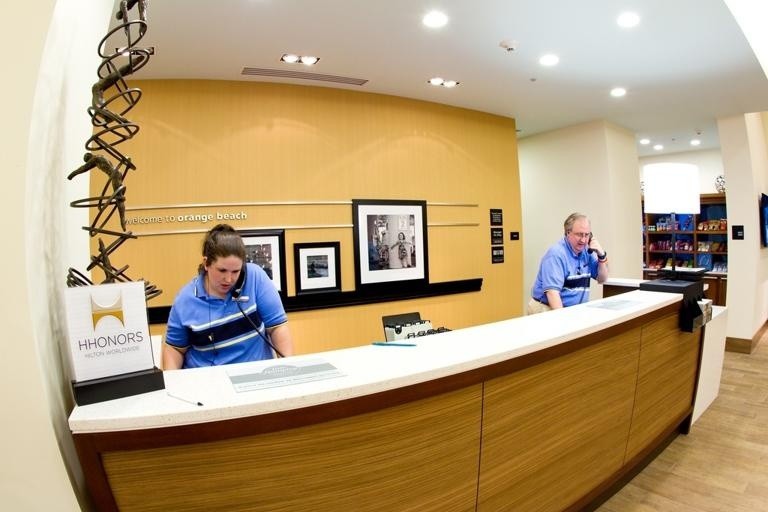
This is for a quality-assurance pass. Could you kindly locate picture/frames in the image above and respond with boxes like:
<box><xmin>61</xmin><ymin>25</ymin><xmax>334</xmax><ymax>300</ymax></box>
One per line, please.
<box><xmin>353</xmin><ymin>196</ymin><xmax>431</xmax><ymax>288</ymax></box>
<box><xmin>292</xmin><ymin>240</ymin><xmax>343</xmax><ymax>296</ymax></box>
<box><xmin>234</xmin><ymin>228</ymin><xmax>289</xmax><ymax>300</ymax></box>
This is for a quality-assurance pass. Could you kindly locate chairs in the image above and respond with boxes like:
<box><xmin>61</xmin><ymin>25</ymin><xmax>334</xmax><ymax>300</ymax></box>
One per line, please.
<box><xmin>382</xmin><ymin>310</ymin><xmax>426</xmax><ymax>344</ymax></box>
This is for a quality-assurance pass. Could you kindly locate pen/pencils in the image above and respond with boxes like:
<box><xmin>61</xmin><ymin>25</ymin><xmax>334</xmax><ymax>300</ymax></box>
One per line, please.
<box><xmin>372</xmin><ymin>342</ymin><xmax>416</xmax><ymax>347</ymax></box>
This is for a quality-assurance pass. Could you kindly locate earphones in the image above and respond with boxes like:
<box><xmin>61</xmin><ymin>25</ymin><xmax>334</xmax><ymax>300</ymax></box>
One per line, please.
<box><xmin>205</xmin><ymin>260</ymin><xmax>209</xmax><ymax>267</ymax></box>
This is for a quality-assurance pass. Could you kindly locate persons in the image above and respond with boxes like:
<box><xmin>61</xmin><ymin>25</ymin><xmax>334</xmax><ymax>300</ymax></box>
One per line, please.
<box><xmin>526</xmin><ymin>213</ymin><xmax>608</xmax><ymax>315</ymax></box>
<box><xmin>390</xmin><ymin>232</ymin><xmax>415</xmax><ymax>268</ymax></box>
<box><xmin>162</xmin><ymin>224</ymin><xmax>293</xmax><ymax>371</ymax></box>
<box><xmin>311</xmin><ymin>260</ymin><xmax>318</xmax><ymax>273</ymax></box>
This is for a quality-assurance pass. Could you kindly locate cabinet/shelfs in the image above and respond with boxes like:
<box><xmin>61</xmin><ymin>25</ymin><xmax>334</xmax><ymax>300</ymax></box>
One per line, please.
<box><xmin>642</xmin><ymin>196</ymin><xmax>727</xmax><ymax>305</ymax></box>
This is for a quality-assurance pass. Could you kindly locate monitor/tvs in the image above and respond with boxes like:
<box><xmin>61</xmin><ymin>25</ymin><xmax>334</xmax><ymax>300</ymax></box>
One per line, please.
<box><xmin>757</xmin><ymin>192</ymin><xmax>768</xmax><ymax>245</ymax></box>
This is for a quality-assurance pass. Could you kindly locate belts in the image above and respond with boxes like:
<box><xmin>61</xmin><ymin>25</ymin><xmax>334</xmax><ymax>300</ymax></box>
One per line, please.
<box><xmin>533</xmin><ymin>296</ymin><xmax>549</xmax><ymax>306</ymax></box>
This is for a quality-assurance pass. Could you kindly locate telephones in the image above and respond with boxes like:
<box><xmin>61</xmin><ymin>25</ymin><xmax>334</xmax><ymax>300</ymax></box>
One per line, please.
<box><xmin>587</xmin><ymin>232</ymin><xmax>593</xmax><ymax>254</ymax></box>
<box><xmin>231</xmin><ymin>264</ymin><xmax>247</xmax><ymax>298</ymax></box>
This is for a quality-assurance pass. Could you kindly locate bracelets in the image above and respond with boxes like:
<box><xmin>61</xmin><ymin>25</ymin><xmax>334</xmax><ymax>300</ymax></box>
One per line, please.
<box><xmin>599</xmin><ymin>259</ymin><xmax>607</xmax><ymax>263</ymax></box>
<box><xmin>597</xmin><ymin>252</ymin><xmax>606</xmax><ymax>259</ymax></box>
